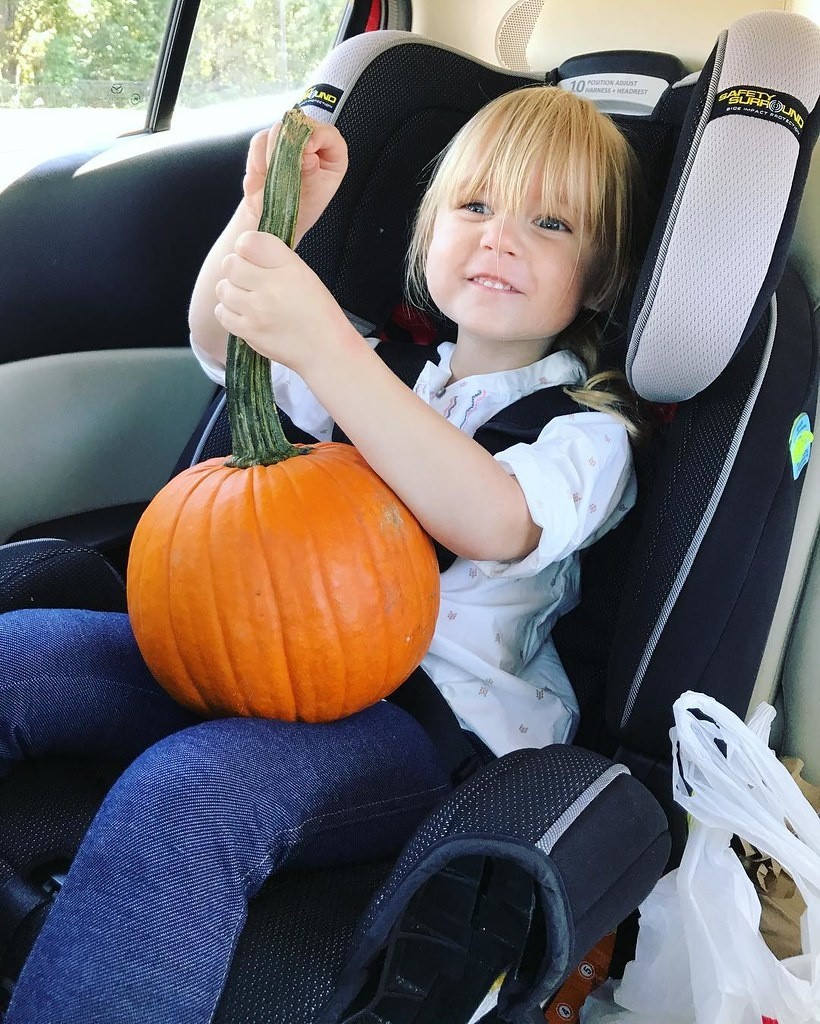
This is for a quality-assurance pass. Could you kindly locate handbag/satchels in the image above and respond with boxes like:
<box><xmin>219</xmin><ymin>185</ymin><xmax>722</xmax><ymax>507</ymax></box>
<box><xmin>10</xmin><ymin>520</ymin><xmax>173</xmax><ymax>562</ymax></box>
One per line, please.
<box><xmin>614</xmin><ymin>689</ymin><xmax>819</xmax><ymax>1024</ymax></box>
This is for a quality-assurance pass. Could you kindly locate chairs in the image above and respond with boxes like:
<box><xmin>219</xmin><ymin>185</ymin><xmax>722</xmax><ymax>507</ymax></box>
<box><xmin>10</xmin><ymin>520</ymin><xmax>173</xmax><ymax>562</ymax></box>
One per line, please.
<box><xmin>0</xmin><ymin>8</ymin><xmax>820</xmax><ymax>1024</ymax></box>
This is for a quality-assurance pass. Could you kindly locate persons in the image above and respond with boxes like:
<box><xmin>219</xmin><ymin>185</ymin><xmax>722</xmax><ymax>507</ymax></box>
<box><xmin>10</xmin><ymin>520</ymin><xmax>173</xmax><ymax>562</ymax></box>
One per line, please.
<box><xmin>0</xmin><ymin>86</ymin><xmax>635</xmax><ymax>1024</ymax></box>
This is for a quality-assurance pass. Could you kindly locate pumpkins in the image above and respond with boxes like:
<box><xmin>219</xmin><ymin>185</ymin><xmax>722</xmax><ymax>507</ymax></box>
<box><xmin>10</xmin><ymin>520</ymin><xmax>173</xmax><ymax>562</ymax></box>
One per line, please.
<box><xmin>127</xmin><ymin>441</ymin><xmax>441</xmax><ymax>723</ymax></box>
